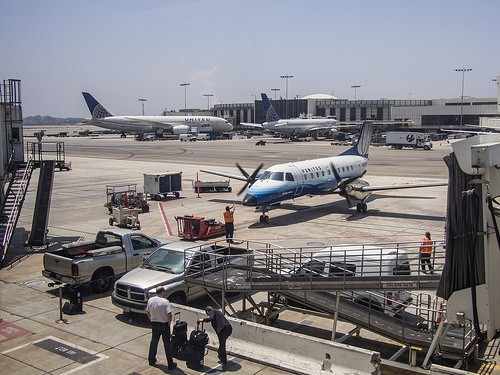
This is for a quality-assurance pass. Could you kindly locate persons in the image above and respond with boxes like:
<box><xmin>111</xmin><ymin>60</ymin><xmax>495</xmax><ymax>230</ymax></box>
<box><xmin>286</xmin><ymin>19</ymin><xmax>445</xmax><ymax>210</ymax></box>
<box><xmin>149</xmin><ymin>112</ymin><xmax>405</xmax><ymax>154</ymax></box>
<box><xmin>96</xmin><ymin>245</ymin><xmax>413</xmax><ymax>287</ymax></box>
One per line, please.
<box><xmin>198</xmin><ymin>306</ymin><xmax>232</xmax><ymax>366</ymax></box>
<box><xmin>419</xmin><ymin>232</ymin><xmax>434</xmax><ymax>273</ymax></box>
<box><xmin>223</xmin><ymin>202</ymin><xmax>236</xmax><ymax>243</ymax></box>
<box><xmin>145</xmin><ymin>285</ymin><xmax>177</xmax><ymax>370</ymax></box>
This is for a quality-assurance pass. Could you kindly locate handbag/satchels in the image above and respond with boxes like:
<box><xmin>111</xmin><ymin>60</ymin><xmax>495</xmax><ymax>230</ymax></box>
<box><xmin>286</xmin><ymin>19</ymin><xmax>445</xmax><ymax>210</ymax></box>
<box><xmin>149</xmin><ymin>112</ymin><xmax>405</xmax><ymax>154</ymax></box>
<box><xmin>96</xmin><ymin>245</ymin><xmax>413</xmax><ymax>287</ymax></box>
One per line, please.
<box><xmin>189</xmin><ymin>329</ymin><xmax>209</xmax><ymax>347</ymax></box>
<box><xmin>62</xmin><ymin>302</ymin><xmax>78</xmax><ymax>315</ymax></box>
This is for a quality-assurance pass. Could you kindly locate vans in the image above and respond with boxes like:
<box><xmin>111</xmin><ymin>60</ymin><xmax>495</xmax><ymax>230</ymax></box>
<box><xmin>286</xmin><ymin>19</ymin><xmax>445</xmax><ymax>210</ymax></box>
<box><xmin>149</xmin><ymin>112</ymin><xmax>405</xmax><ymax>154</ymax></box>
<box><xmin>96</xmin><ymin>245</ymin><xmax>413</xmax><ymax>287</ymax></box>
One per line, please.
<box><xmin>270</xmin><ymin>243</ymin><xmax>413</xmax><ymax>318</ymax></box>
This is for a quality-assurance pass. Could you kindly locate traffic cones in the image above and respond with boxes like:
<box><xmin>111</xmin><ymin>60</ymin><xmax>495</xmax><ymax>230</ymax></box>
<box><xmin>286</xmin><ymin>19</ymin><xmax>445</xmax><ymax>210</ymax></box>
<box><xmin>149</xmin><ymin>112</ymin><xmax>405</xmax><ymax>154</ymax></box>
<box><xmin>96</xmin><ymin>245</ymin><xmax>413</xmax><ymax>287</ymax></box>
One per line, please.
<box><xmin>437</xmin><ymin>303</ymin><xmax>443</xmax><ymax>323</ymax></box>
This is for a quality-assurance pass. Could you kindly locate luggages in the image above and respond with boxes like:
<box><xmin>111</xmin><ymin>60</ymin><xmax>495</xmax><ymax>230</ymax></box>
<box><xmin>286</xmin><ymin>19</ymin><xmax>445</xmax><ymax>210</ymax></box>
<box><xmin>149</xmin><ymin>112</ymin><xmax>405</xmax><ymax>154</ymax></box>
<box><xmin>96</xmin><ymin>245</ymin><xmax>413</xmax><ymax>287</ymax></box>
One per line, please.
<box><xmin>186</xmin><ymin>319</ymin><xmax>205</xmax><ymax>372</ymax></box>
<box><xmin>69</xmin><ymin>277</ymin><xmax>82</xmax><ymax>314</ymax></box>
<box><xmin>169</xmin><ymin>312</ymin><xmax>188</xmax><ymax>361</ymax></box>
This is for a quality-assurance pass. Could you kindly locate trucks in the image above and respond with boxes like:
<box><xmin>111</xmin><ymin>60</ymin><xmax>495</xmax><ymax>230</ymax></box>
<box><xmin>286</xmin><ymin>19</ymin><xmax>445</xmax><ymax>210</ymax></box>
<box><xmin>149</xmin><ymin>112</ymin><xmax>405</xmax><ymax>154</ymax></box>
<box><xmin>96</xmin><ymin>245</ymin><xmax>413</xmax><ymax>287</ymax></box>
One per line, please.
<box><xmin>180</xmin><ymin>134</ymin><xmax>196</xmax><ymax>142</ymax></box>
<box><xmin>386</xmin><ymin>131</ymin><xmax>433</xmax><ymax>151</ymax></box>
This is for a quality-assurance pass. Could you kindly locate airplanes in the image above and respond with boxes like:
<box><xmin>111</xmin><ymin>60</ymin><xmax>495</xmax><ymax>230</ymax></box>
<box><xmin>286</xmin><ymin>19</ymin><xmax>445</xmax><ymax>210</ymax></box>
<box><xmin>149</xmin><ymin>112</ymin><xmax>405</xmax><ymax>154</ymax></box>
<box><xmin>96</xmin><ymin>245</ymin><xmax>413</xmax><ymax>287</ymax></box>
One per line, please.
<box><xmin>238</xmin><ymin>92</ymin><xmax>358</xmax><ymax>141</ymax></box>
<box><xmin>199</xmin><ymin>119</ymin><xmax>450</xmax><ymax>225</ymax></box>
<box><xmin>81</xmin><ymin>91</ymin><xmax>234</xmax><ymax>142</ymax></box>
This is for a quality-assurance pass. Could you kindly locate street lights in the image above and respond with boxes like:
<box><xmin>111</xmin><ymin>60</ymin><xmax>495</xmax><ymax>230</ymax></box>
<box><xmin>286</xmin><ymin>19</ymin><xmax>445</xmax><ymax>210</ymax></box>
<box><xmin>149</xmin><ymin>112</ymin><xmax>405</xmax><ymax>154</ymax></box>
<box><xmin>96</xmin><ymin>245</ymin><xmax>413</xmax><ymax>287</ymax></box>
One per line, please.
<box><xmin>203</xmin><ymin>95</ymin><xmax>214</xmax><ymax>111</ymax></box>
<box><xmin>180</xmin><ymin>83</ymin><xmax>191</xmax><ymax>116</ymax></box>
<box><xmin>351</xmin><ymin>85</ymin><xmax>360</xmax><ymax>100</ymax></box>
<box><xmin>455</xmin><ymin>69</ymin><xmax>473</xmax><ymax>130</ymax></box>
<box><xmin>280</xmin><ymin>76</ymin><xmax>293</xmax><ymax>120</ymax></box>
<box><xmin>271</xmin><ymin>88</ymin><xmax>280</xmax><ymax>100</ymax></box>
<box><xmin>138</xmin><ymin>98</ymin><xmax>147</xmax><ymax>116</ymax></box>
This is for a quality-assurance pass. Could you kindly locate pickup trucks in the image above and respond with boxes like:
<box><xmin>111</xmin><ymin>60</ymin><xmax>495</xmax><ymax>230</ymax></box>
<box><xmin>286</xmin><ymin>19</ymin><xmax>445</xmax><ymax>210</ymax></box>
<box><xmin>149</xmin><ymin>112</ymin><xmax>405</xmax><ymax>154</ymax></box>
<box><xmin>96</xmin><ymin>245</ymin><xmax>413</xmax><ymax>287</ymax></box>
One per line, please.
<box><xmin>111</xmin><ymin>242</ymin><xmax>253</xmax><ymax>316</ymax></box>
<box><xmin>41</xmin><ymin>228</ymin><xmax>177</xmax><ymax>295</ymax></box>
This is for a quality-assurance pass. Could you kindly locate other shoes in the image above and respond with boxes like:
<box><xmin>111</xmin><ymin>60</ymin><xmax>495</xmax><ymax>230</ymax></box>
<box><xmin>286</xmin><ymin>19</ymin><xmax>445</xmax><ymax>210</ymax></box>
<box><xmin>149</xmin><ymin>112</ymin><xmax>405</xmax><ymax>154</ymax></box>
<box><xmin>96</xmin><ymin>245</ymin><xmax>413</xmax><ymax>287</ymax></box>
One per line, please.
<box><xmin>226</xmin><ymin>241</ymin><xmax>230</xmax><ymax>243</ymax></box>
<box><xmin>230</xmin><ymin>241</ymin><xmax>234</xmax><ymax>243</ymax></box>
<box><xmin>217</xmin><ymin>361</ymin><xmax>227</xmax><ymax>364</ymax></box>
<box><xmin>218</xmin><ymin>350</ymin><xmax>221</xmax><ymax>359</ymax></box>
<box><xmin>149</xmin><ymin>358</ymin><xmax>157</xmax><ymax>366</ymax></box>
<box><xmin>168</xmin><ymin>362</ymin><xmax>177</xmax><ymax>370</ymax></box>
